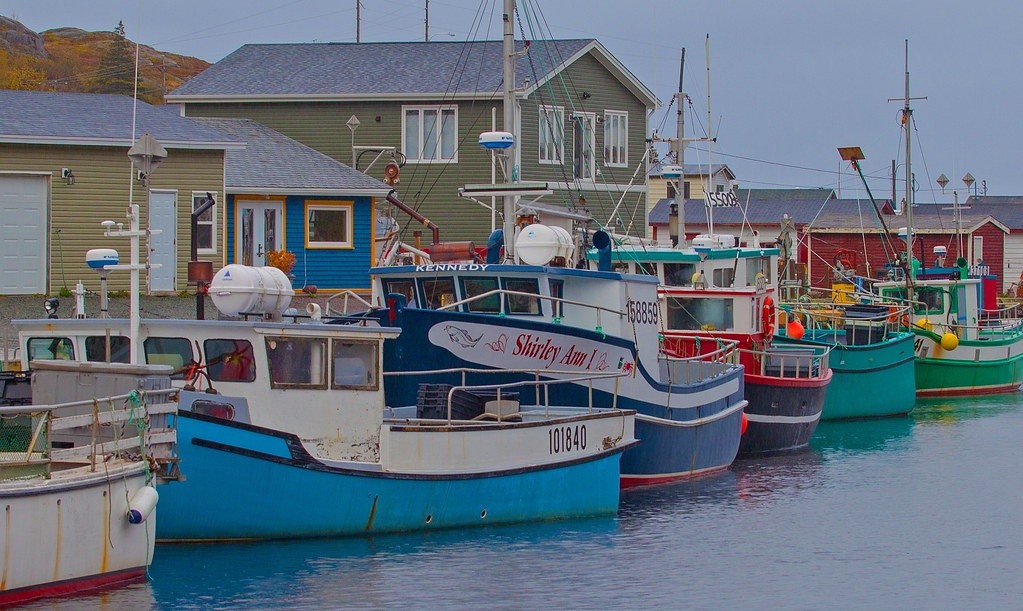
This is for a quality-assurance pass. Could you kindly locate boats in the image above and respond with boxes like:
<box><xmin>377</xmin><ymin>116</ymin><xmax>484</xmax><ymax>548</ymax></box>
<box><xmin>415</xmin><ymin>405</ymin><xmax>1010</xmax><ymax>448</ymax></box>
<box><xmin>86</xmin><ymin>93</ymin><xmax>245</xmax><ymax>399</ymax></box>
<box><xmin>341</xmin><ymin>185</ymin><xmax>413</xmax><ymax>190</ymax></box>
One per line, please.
<box><xmin>322</xmin><ymin>0</ymin><xmax>833</xmax><ymax>493</ymax></box>
<box><xmin>0</xmin><ymin>41</ymin><xmax>640</xmax><ymax>539</ymax></box>
<box><xmin>0</xmin><ymin>360</ymin><xmax>180</xmax><ymax>607</ymax></box>
<box><xmin>577</xmin><ymin>33</ymin><xmax>1023</xmax><ymax>421</ymax></box>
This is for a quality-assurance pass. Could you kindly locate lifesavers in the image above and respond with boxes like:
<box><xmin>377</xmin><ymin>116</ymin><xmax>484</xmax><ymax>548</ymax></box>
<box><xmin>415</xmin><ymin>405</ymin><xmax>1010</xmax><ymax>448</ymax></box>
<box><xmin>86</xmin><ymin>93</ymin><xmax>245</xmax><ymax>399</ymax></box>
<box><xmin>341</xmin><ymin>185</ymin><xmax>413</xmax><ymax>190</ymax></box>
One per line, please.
<box><xmin>887</xmin><ymin>307</ymin><xmax>909</xmax><ymax>326</ymax></box>
<box><xmin>763</xmin><ymin>296</ymin><xmax>775</xmax><ymax>338</ymax></box>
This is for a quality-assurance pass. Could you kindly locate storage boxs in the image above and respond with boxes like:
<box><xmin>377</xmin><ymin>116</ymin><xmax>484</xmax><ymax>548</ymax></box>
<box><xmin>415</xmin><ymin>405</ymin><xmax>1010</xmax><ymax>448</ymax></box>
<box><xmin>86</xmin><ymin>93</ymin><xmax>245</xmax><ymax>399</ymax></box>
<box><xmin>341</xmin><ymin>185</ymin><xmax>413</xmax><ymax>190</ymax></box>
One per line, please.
<box><xmin>415</xmin><ymin>383</ymin><xmax>523</xmax><ymax>422</ymax></box>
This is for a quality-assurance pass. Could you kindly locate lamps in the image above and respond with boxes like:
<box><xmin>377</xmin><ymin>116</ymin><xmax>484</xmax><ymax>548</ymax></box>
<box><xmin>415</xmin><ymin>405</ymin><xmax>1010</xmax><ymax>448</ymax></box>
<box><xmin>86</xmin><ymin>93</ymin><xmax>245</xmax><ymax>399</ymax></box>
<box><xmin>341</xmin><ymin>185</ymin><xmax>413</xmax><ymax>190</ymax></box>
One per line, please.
<box><xmin>137</xmin><ymin>169</ymin><xmax>147</xmax><ymax>187</ymax></box>
<box><xmin>597</xmin><ymin>115</ymin><xmax>606</xmax><ymax>125</ymax></box>
<box><xmin>61</xmin><ymin>167</ymin><xmax>76</xmax><ymax>185</ymax></box>
